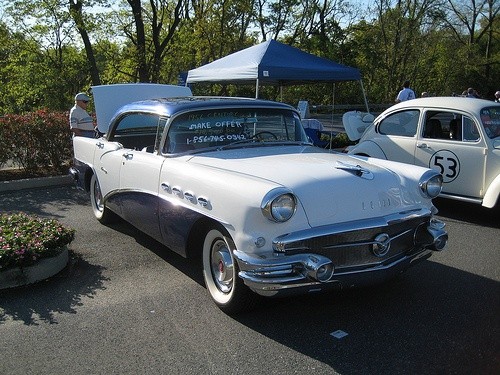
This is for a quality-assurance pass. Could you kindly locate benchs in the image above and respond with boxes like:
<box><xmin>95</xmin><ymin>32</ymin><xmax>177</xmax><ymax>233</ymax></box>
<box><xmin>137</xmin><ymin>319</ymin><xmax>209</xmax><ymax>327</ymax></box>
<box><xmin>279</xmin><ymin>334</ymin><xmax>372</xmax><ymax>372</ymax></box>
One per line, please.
<box><xmin>114</xmin><ymin>133</ymin><xmax>190</xmax><ymax>150</ymax></box>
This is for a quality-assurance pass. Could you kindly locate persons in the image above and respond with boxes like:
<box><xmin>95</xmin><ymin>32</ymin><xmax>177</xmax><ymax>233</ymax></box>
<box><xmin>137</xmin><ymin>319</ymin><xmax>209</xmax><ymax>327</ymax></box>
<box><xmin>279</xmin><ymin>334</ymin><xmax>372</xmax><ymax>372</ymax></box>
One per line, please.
<box><xmin>69</xmin><ymin>92</ymin><xmax>94</xmax><ymax>190</ymax></box>
<box><xmin>452</xmin><ymin>88</ymin><xmax>500</xmax><ymax>114</ymax></box>
<box><xmin>395</xmin><ymin>81</ymin><xmax>415</xmax><ymax>103</ymax></box>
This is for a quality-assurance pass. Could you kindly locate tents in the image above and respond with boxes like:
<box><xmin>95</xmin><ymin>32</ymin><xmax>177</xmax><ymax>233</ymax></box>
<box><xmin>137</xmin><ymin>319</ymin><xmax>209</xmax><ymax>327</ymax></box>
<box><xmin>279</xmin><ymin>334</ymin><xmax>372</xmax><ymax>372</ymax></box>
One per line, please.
<box><xmin>184</xmin><ymin>38</ymin><xmax>371</xmax><ymax>150</ymax></box>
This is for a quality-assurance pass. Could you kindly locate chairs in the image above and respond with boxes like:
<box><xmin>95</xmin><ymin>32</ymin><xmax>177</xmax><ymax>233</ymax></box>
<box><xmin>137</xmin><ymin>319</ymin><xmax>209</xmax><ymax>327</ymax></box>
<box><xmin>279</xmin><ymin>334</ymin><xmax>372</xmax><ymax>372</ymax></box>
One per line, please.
<box><xmin>301</xmin><ymin>119</ymin><xmax>331</xmax><ymax>149</ymax></box>
<box><xmin>449</xmin><ymin>119</ymin><xmax>462</xmax><ymax>139</ymax></box>
<box><xmin>425</xmin><ymin>119</ymin><xmax>444</xmax><ymax>137</ymax></box>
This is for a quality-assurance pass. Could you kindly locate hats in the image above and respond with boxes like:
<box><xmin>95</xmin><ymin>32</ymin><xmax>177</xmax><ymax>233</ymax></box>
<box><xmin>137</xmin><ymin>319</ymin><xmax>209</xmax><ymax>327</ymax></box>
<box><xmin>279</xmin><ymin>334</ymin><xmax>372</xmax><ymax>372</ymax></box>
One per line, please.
<box><xmin>401</xmin><ymin>81</ymin><xmax>410</xmax><ymax>87</ymax></box>
<box><xmin>75</xmin><ymin>93</ymin><xmax>91</xmax><ymax>101</ymax></box>
<box><xmin>468</xmin><ymin>87</ymin><xmax>472</xmax><ymax>91</ymax></box>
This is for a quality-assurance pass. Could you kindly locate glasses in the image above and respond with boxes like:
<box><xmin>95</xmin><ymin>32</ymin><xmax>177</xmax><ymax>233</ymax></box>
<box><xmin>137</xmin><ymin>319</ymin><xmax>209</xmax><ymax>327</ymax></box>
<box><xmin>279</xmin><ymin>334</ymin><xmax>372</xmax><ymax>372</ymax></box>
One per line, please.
<box><xmin>83</xmin><ymin>101</ymin><xmax>88</xmax><ymax>103</ymax></box>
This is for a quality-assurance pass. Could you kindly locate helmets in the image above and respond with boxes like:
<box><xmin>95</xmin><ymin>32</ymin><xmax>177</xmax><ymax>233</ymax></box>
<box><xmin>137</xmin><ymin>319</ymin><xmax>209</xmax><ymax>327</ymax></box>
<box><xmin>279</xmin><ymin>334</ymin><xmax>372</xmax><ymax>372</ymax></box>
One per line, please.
<box><xmin>422</xmin><ymin>92</ymin><xmax>428</xmax><ymax>97</ymax></box>
<box><xmin>495</xmin><ymin>91</ymin><xmax>500</xmax><ymax>95</ymax></box>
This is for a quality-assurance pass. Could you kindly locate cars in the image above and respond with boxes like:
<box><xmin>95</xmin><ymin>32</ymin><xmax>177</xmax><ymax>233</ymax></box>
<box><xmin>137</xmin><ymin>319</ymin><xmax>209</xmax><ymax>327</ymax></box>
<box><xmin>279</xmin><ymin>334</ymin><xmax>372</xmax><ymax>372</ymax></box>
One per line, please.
<box><xmin>70</xmin><ymin>83</ymin><xmax>448</xmax><ymax>313</ymax></box>
<box><xmin>346</xmin><ymin>96</ymin><xmax>500</xmax><ymax>228</ymax></box>
